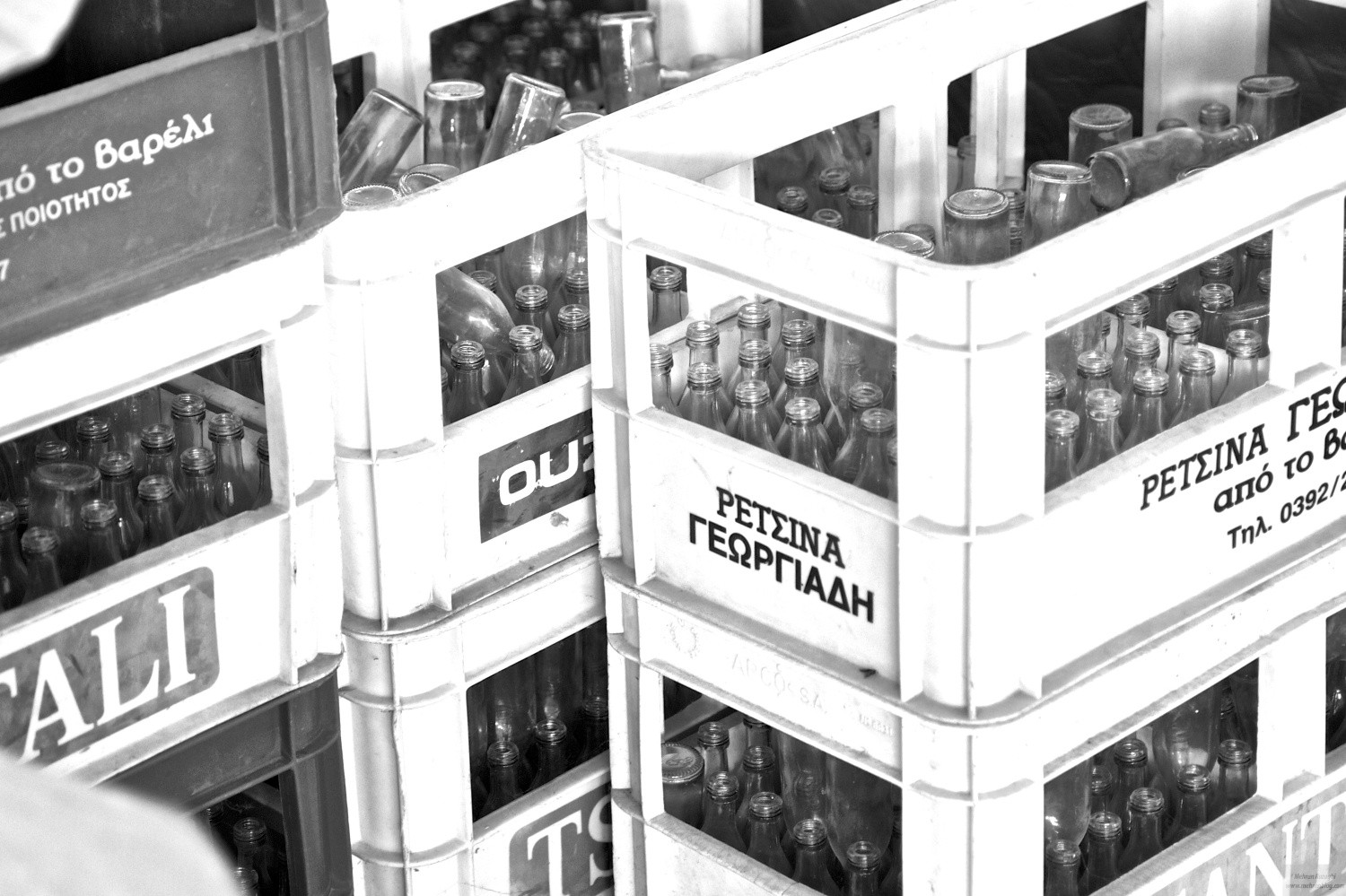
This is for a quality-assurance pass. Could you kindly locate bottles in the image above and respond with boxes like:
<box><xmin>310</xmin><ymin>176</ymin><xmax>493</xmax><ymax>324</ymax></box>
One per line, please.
<box><xmin>1</xmin><ymin>1</ymin><xmax>1345</xmax><ymax>896</ymax></box>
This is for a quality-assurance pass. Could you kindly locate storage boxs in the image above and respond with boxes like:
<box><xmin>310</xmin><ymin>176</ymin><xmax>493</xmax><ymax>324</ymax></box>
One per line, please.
<box><xmin>0</xmin><ymin>0</ymin><xmax>1346</xmax><ymax>896</ymax></box>
